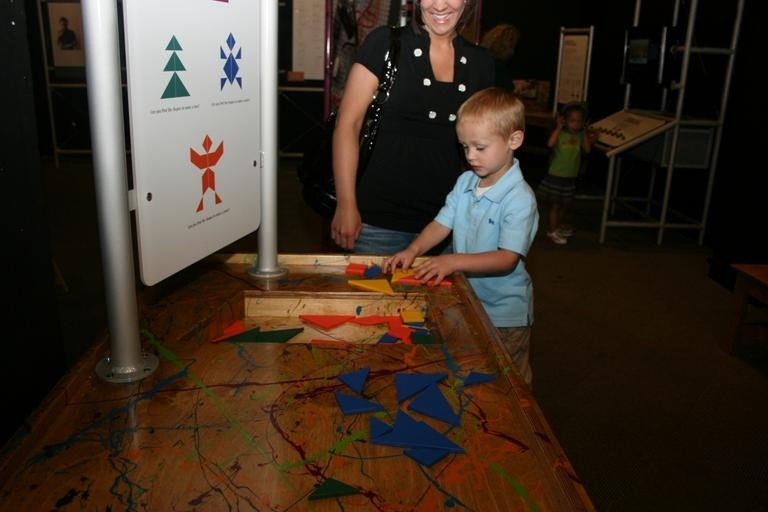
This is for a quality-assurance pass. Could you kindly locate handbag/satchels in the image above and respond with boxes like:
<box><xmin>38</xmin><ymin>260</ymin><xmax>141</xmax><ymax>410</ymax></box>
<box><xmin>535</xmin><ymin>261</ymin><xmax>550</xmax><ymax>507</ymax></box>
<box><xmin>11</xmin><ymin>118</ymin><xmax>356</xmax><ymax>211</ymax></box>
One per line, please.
<box><xmin>321</xmin><ymin>99</ymin><xmax>380</xmax><ymax>203</ymax></box>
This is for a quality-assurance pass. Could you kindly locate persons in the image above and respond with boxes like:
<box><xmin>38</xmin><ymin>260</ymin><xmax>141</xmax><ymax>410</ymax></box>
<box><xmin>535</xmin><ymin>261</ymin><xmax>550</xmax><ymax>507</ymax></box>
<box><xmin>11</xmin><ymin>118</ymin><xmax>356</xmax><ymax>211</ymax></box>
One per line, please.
<box><xmin>381</xmin><ymin>88</ymin><xmax>539</xmax><ymax>391</ymax></box>
<box><xmin>546</xmin><ymin>105</ymin><xmax>591</xmax><ymax>244</ymax></box>
<box><xmin>331</xmin><ymin>1</ymin><xmax>499</xmax><ymax>256</ymax></box>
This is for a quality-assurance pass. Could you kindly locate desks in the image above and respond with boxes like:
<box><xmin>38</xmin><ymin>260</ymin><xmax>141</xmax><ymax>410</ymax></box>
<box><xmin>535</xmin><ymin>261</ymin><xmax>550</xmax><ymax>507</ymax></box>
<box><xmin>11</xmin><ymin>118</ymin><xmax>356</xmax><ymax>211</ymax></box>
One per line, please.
<box><xmin>0</xmin><ymin>252</ymin><xmax>592</xmax><ymax>510</ymax></box>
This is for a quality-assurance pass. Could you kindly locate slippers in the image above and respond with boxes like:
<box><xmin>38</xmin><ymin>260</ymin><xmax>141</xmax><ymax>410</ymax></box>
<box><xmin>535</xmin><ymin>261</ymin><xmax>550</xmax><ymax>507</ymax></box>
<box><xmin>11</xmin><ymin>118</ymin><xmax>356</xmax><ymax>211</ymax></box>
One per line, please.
<box><xmin>549</xmin><ymin>229</ymin><xmax>569</xmax><ymax>247</ymax></box>
